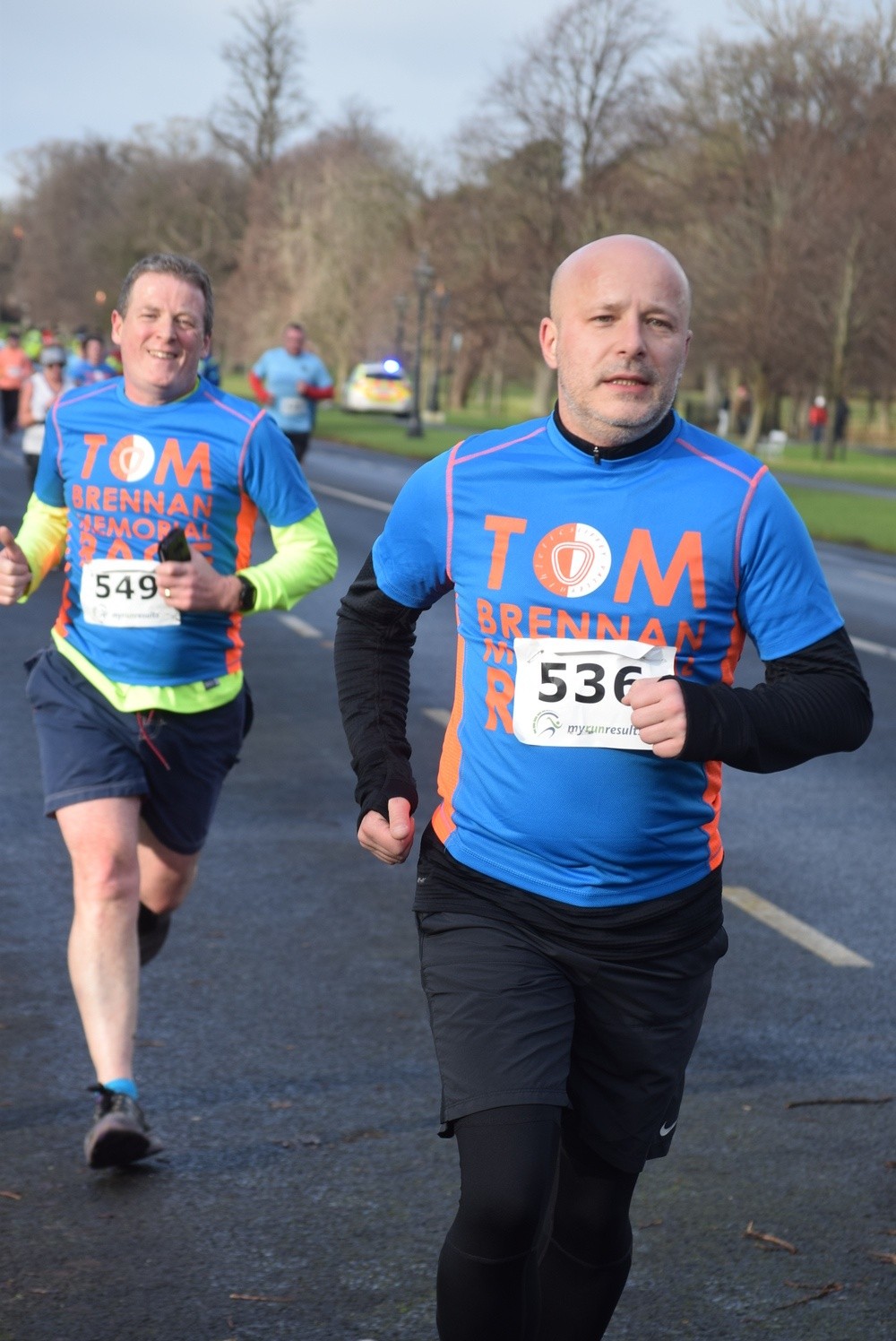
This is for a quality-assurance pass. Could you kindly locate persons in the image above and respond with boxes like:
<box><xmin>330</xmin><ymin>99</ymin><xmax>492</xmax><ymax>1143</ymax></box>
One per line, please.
<box><xmin>715</xmin><ymin>386</ymin><xmax>752</xmax><ymax>437</ymax></box>
<box><xmin>0</xmin><ymin>310</ymin><xmax>125</xmax><ymax>500</ymax></box>
<box><xmin>0</xmin><ymin>251</ymin><xmax>336</xmax><ymax>1168</ymax></box>
<box><xmin>809</xmin><ymin>394</ymin><xmax>852</xmax><ymax>462</ymax></box>
<box><xmin>334</xmin><ymin>233</ymin><xmax>875</xmax><ymax>1341</ymax></box>
<box><xmin>199</xmin><ymin>356</ymin><xmax>220</xmax><ymax>389</ymax></box>
<box><xmin>246</xmin><ymin>323</ymin><xmax>335</xmax><ymax>466</ymax></box>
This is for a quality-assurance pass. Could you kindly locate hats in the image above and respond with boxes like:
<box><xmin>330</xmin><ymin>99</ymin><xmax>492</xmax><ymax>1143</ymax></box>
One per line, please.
<box><xmin>40</xmin><ymin>347</ymin><xmax>67</xmax><ymax>364</ymax></box>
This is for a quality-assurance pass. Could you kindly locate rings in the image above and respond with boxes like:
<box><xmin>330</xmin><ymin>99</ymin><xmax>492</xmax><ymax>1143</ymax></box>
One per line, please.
<box><xmin>165</xmin><ymin>587</ymin><xmax>170</xmax><ymax>599</ymax></box>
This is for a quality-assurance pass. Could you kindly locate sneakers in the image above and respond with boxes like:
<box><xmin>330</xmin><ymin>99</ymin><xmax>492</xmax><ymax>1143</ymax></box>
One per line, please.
<box><xmin>84</xmin><ymin>1084</ymin><xmax>166</xmax><ymax>1172</ymax></box>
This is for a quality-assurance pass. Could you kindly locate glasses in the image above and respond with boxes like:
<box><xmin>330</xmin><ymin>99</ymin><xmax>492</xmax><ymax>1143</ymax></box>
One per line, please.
<box><xmin>48</xmin><ymin>364</ymin><xmax>65</xmax><ymax>368</ymax></box>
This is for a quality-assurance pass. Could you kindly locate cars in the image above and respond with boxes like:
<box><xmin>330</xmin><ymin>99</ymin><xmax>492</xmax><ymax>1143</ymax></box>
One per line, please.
<box><xmin>338</xmin><ymin>357</ymin><xmax>416</xmax><ymax>416</ymax></box>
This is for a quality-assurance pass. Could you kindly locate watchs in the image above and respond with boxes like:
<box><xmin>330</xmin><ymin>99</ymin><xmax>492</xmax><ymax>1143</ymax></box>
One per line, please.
<box><xmin>237</xmin><ymin>574</ymin><xmax>257</xmax><ymax>611</ymax></box>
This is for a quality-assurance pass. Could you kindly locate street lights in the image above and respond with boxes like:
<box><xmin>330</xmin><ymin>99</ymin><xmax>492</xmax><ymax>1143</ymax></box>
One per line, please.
<box><xmin>408</xmin><ymin>261</ymin><xmax>437</xmax><ymax>442</ymax></box>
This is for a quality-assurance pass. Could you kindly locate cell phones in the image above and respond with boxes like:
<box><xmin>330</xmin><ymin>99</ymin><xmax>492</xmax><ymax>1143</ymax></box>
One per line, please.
<box><xmin>159</xmin><ymin>527</ymin><xmax>191</xmax><ymax>562</ymax></box>
